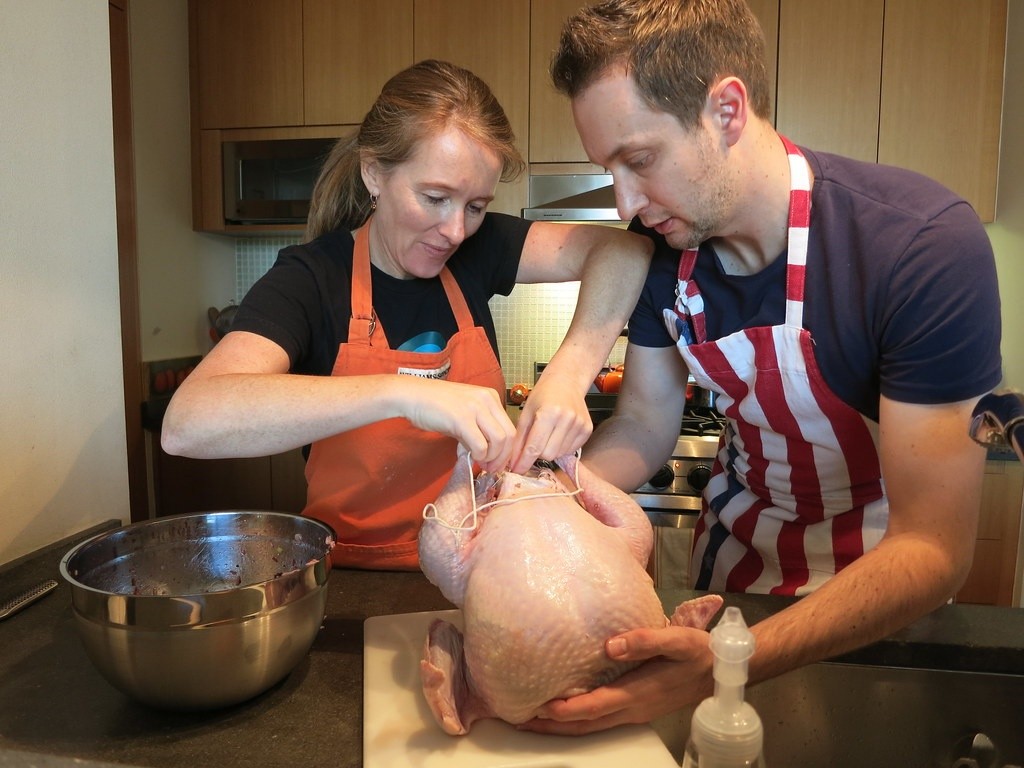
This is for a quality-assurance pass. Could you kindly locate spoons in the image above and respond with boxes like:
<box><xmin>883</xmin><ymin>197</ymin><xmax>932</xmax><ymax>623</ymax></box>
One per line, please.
<box><xmin>0</xmin><ymin>577</ymin><xmax>59</xmax><ymax>620</ymax></box>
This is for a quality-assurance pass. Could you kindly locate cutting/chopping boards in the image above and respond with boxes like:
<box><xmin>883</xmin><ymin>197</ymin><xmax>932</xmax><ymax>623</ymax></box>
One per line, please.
<box><xmin>360</xmin><ymin>607</ymin><xmax>680</xmax><ymax>768</ymax></box>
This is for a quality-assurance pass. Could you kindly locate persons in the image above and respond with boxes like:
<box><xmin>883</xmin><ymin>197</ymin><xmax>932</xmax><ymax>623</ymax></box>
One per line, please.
<box><xmin>521</xmin><ymin>0</ymin><xmax>1005</xmax><ymax>737</ymax></box>
<box><xmin>161</xmin><ymin>60</ymin><xmax>657</xmax><ymax>571</ymax></box>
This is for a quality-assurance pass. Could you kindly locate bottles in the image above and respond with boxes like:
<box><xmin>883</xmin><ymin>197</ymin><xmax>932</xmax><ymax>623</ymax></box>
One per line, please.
<box><xmin>680</xmin><ymin>604</ymin><xmax>766</xmax><ymax>768</ymax></box>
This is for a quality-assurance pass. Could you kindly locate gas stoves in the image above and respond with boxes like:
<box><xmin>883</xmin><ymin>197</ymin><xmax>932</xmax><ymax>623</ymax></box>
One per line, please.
<box><xmin>590</xmin><ymin>408</ymin><xmax>729</xmax><ymax>514</ymax></box>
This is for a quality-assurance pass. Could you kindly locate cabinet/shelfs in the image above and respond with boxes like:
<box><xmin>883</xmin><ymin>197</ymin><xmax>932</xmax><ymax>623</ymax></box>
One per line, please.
<box><xmin>156</xmin><ymin>453</ymin><xmax>1022</xmax><ymax>607</ymax></box>
<box><xmin>185</xmin><ymin>0</ymin><xmax>1010</xmax><ymax>240</ymax></box>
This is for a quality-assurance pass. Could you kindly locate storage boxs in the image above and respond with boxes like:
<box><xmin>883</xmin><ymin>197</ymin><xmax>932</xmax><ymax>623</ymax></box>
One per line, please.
<box><xmin>59</xmin><ymin>509</ymin><xmax>337</xmax><ymax>715</ymax></box>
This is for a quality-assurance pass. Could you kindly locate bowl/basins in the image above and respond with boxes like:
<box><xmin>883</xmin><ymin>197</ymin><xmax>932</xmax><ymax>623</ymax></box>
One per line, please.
<box><xmin>689</xmin><ymin>384</ymin><xmax>720</xmax><ymax>410</ymax></box>
<box><xmin>59</xmin><ymin>507</ymin><xmax>340</xmax><ymax>714</ymax></box>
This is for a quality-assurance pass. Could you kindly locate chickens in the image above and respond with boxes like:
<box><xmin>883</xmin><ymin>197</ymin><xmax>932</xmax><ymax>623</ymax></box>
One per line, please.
<box><xmin>419</xmin><ymin>445</ymin><xmax>724</xmax><ymax>736</ymax></box>
